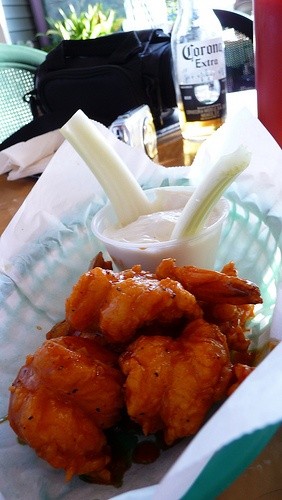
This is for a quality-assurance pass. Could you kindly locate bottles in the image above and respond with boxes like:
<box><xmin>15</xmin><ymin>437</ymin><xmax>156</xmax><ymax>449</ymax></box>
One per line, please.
<box><xmin>170</xmin><ymin>0</ymin><xmax>227</xmax><ymax>144</ymax></box>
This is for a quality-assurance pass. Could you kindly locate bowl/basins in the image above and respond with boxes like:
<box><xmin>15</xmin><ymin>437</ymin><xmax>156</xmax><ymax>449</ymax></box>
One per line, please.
<box><xmin>92</xmin><ymin>185</ymin><xmax>233</xmax><ymax>270</ymax></box>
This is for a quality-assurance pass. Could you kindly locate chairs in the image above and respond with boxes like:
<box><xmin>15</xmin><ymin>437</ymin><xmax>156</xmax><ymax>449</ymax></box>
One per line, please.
<box><xmin>0</xmin><ymin>44</ymin><xmax>49</xmax><ymax>146</ymax></box>
<box><xmin>214</xmin><ymin>7</ymin><xmax>255</xmax><ymax>94</ymax></box>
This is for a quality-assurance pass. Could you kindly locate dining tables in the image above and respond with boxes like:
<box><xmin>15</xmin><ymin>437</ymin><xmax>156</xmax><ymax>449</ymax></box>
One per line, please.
<box><xmin>0</xmin><ymin>89</ymin><xmax>282</xmax><ymax>500</ymax></box>
